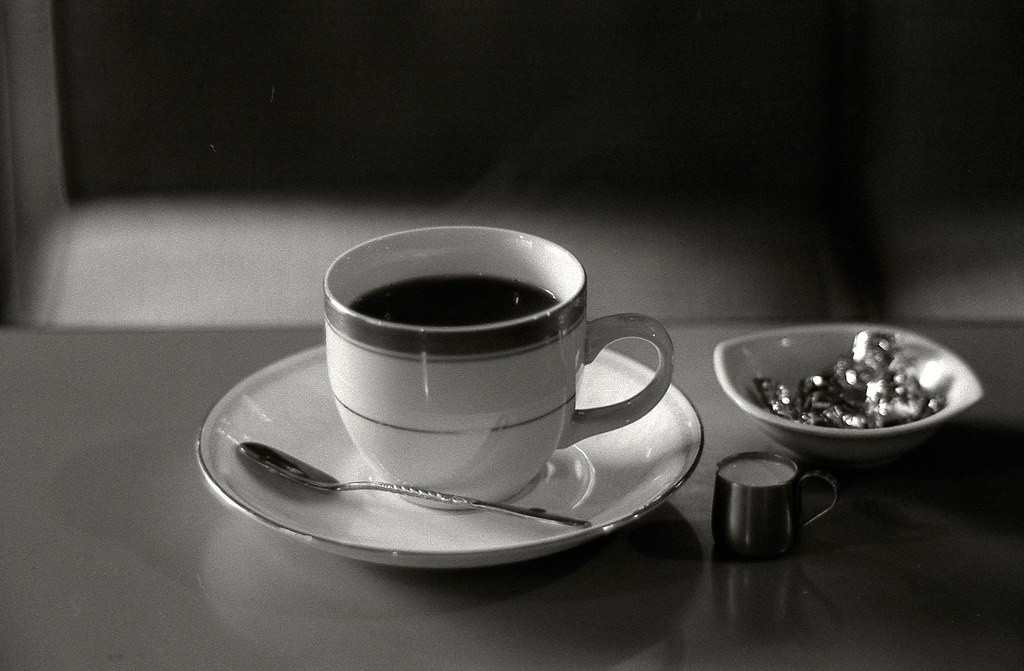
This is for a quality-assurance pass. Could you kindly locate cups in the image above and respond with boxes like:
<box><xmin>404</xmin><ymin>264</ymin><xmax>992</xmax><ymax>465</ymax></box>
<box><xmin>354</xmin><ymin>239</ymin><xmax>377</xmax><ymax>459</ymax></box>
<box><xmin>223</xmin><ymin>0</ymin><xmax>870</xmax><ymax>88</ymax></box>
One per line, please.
<box><xmin>323</xmin><ymin>225</ymin><xmax>676</xmax><ymax>510</ymax></box>
<box><xmin>711</xmin><ymin>452</ymin><xmax>840</xmax><ymax>555</ymax></box>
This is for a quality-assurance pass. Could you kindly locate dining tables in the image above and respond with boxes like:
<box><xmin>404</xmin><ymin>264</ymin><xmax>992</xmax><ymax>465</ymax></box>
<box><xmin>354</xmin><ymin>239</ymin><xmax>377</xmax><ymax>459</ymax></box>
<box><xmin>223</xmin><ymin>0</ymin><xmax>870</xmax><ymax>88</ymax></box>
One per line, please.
<box><xmin>0</xmin><ymin>316</ymin><xmax>1024</xmax><ymax>671</ymax></box>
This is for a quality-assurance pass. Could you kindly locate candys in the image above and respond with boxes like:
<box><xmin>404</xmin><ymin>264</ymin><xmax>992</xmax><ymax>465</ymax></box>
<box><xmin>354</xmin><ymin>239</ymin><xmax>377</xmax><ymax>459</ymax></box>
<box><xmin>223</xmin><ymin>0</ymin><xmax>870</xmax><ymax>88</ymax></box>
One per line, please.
<box><xmin>750</xmin><ymin>330</ymin><xmax>935</xmax><ymax>427</ymax></box>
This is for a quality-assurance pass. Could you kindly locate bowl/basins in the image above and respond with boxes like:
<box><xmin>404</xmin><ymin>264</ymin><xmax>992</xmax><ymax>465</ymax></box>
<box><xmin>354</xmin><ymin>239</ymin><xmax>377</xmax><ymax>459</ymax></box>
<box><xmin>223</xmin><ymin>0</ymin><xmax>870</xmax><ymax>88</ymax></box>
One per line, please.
<box><xmin>713</xmin><ymin>323</ymin><xmax>985</xmax><ymax>460</ymax></box>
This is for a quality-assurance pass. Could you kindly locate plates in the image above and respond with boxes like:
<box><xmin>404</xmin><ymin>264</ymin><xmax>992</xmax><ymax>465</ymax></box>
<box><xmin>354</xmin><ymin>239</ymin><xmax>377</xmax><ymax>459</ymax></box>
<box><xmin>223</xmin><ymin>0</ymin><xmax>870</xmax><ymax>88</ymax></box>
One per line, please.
<box><xmin>195</xmin><ymin>343</ymin><xmax>704</xmax><ymax>568</ymax></box>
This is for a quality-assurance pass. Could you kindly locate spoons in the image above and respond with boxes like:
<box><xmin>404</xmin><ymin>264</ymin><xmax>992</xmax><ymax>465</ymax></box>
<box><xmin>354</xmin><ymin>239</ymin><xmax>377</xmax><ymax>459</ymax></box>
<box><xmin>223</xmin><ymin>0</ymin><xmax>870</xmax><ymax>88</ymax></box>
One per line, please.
<box><xmin>240</xmin><ymin>441</ymin><xmax>592</xmax><ymax>529</ymax></box>
